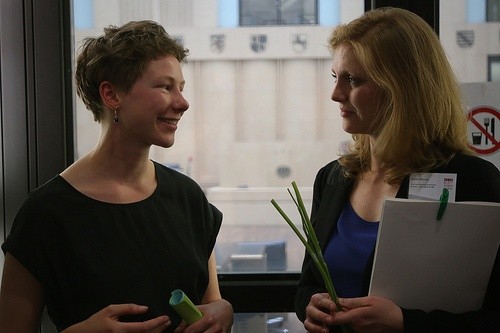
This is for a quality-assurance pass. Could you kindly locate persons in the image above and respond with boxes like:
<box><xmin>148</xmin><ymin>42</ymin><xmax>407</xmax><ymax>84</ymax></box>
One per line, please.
<box><xmin>0</xmin><ymin>20</ymin><xmax>235</xmax><ymax>333</ymax></box>
<box><xmin>297</xmin><ymin>7</ymin><xmax>500</xmax><ymax>333</ymax></box>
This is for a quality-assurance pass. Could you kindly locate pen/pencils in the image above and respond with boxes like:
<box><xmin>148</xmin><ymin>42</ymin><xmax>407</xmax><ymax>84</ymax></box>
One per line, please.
<box><xmin>435</xmin><ymin>187</ymin><xmax>449</xmax><ymax>223</ymax></box>
